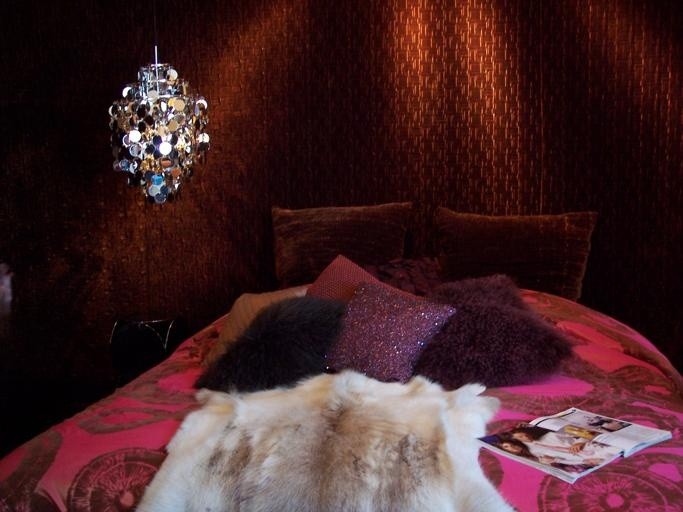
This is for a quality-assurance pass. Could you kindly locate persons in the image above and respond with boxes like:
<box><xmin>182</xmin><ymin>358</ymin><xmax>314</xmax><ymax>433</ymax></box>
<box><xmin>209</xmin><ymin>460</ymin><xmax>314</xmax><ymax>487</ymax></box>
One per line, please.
<box><xmin>491</xmin><ymin>427</ymin><xmax>614</xmax><ymax>474</ymax></box>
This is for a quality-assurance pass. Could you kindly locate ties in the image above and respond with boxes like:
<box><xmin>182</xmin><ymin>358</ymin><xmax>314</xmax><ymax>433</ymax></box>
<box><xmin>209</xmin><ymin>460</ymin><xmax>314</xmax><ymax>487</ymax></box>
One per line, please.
<box><xmin>535</xmin><ymin>443</ymin><xmax>594</xmax><ymax>455</ymax></box>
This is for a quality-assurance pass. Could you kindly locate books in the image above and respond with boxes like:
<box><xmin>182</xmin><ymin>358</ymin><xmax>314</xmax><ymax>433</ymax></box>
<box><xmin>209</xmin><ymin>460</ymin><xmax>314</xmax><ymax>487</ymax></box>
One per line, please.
<box><xmin>475</xmin><ymin>408</ymin><xmax>673</xmax><ymax>485</ymax></box>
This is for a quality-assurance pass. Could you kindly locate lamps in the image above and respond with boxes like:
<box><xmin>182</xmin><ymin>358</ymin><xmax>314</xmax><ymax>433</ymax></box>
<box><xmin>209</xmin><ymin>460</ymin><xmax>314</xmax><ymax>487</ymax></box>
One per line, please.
<box><xmin>104</xmin><ymin>44</ymin><xmax>211</xmax><ymax>205</ymax></box>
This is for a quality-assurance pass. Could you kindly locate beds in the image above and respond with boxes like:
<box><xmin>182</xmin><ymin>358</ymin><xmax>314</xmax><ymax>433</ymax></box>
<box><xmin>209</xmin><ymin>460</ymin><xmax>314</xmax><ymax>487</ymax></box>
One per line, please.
<box><xmin>0</xmin><ymin>283</ymin><xmax>683</xmax><ymax>511</ymax></box>
